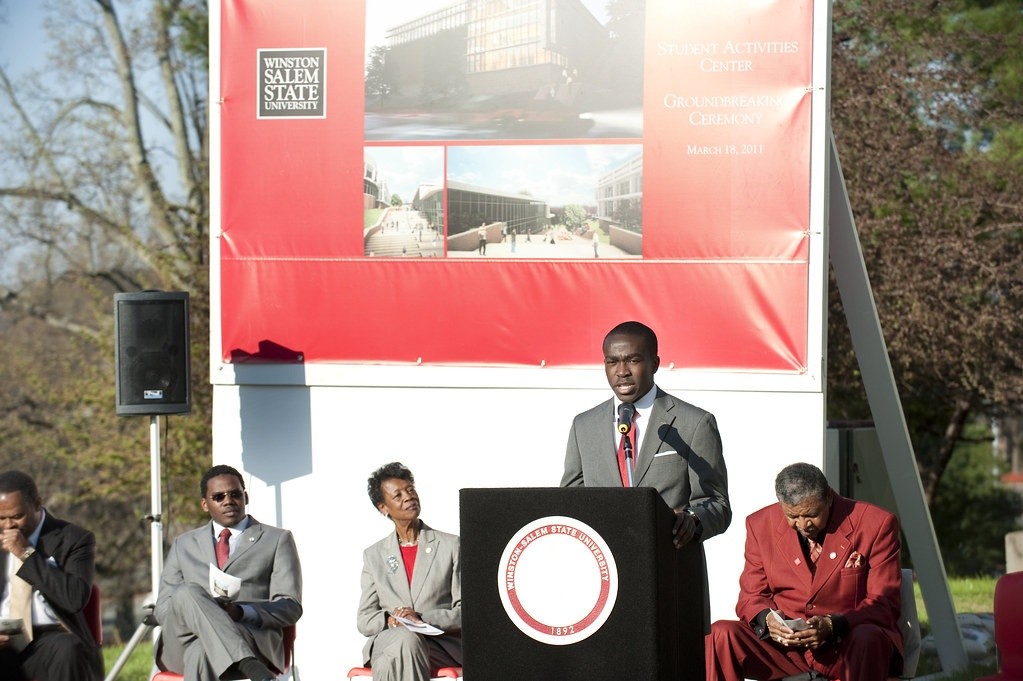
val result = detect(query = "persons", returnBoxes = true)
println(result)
[0,472,108,681]
[707,463,905,680]
[154,464,303,681]
[357,462,462,680]
[561,321,732,638]
[373,203,565,254]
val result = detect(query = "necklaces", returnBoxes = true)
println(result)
[396,537,419,547]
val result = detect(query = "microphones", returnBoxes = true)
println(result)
[618,401,636,434]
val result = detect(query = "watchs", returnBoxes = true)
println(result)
[20,549,36,562]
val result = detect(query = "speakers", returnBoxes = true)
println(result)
[114,292,191,416]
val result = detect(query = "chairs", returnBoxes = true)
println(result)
[348,665,463,681]
[141,602,296,680]
[971,569,1023,680]
[82,586,103,653]
[778,569,921,680]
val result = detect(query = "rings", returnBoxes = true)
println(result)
[398,606,403,612]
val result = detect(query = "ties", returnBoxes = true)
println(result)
[216,529,232,570]
[617,421,636,487]
[808,537,823,576]
[9,555,33,654]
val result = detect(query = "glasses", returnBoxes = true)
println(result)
[206,489,246,502]
